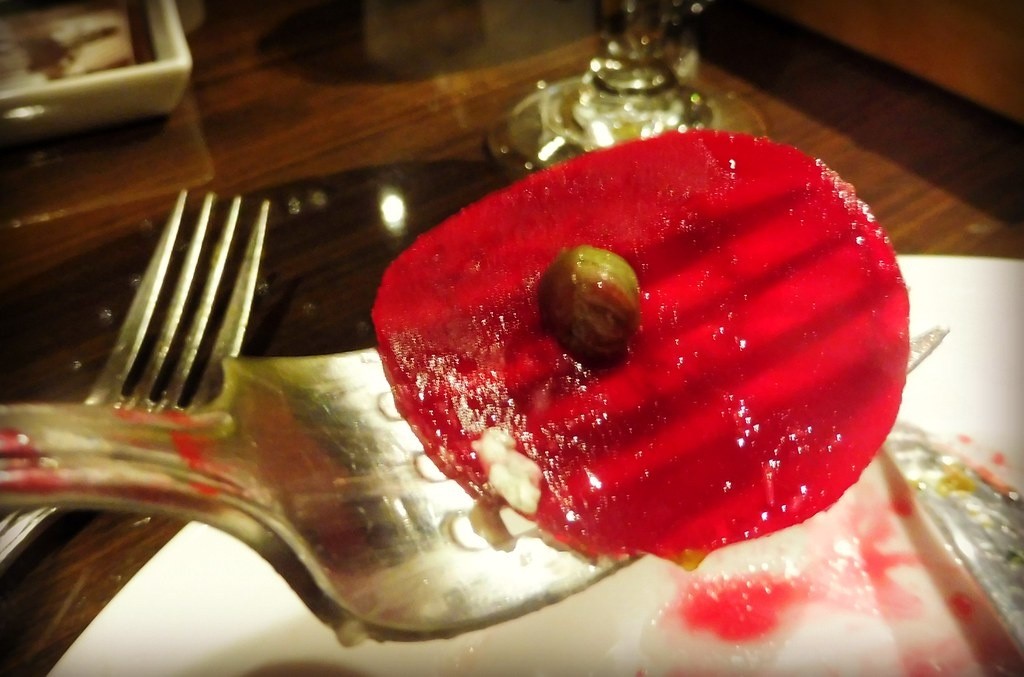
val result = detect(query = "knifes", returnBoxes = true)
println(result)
[877,422,1024,656]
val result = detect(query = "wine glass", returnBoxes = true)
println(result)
[482,0,775,185]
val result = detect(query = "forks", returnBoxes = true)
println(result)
[0,190,272,576]
[0,184,949,644]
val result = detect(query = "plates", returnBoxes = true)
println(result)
[44,254,1024,677]
